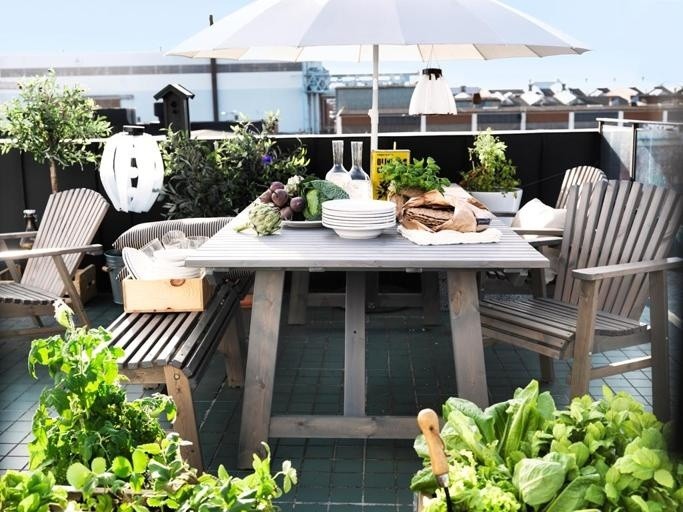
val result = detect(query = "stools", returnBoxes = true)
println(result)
[79,281,247,481]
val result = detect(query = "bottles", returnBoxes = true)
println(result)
[327,138,372,199]
[19,209,39,275]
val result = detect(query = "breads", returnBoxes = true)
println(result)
[386,184,454,229]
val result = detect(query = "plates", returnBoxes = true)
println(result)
[120,242,200,281]
[319,196,397,241]
[286,220,332,229]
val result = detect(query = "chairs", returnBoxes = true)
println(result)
[481,167,605,381]
[481,180,683,428]
[0,187,109,343]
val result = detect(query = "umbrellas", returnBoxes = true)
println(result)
[165,1,595,149]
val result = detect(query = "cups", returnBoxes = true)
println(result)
[140,230,213,271]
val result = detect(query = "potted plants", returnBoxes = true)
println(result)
[461,126,524,228]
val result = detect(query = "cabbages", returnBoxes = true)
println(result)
[292,176,350,222]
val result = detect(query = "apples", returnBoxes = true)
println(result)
[249,181,304,221]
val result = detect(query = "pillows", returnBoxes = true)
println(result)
[111,216,236,254]
[115,267,257,281]
